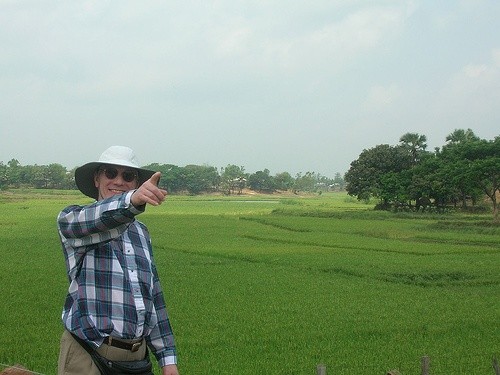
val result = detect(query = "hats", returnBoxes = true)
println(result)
[75,146,160,202]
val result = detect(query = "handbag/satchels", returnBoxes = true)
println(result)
[64,326,152,375]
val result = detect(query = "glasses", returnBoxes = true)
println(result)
[101,167,138,182]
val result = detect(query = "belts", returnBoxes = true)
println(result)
[103,336,144,352]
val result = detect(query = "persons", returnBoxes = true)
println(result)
[56,146,179,375]
